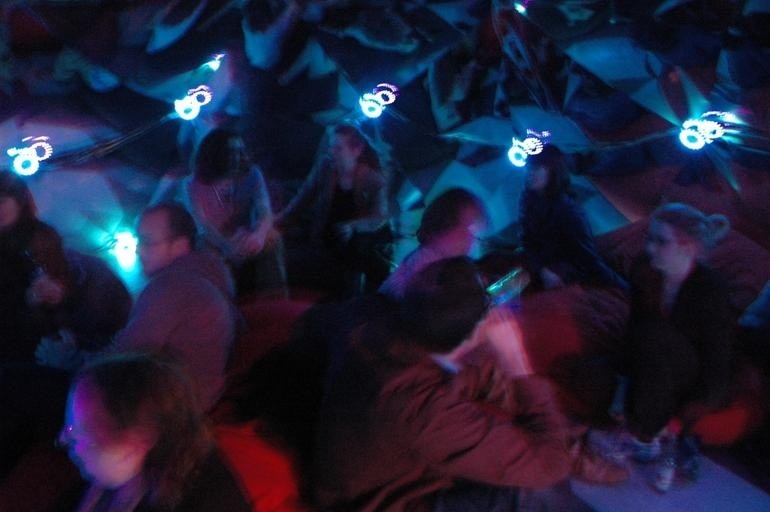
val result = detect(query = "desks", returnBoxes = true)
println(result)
[567,442,770,511]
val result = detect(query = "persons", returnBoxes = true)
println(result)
[0,122,745,512]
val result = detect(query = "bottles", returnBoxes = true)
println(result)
[484,266,530,310]
[649,433,678,494]
